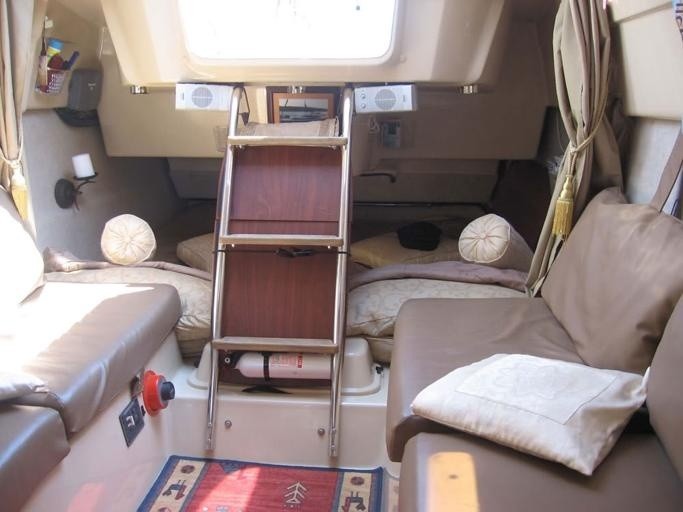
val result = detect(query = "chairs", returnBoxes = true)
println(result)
[384,184,680,464]
[0,182,185,438]
[0,396,72,512]
[398,293,681,512]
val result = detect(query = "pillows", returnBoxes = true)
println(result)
[457,213,532,273]
[96,212,157,266]
[408,350,652,480]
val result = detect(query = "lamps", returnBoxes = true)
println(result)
[54,151,98,212]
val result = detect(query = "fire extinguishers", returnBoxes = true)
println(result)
[224,354,332,379]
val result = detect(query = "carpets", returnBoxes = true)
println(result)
[135,455,386,512]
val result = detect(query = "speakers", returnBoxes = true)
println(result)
[354,84,412,114]
[176,83,233,111]
